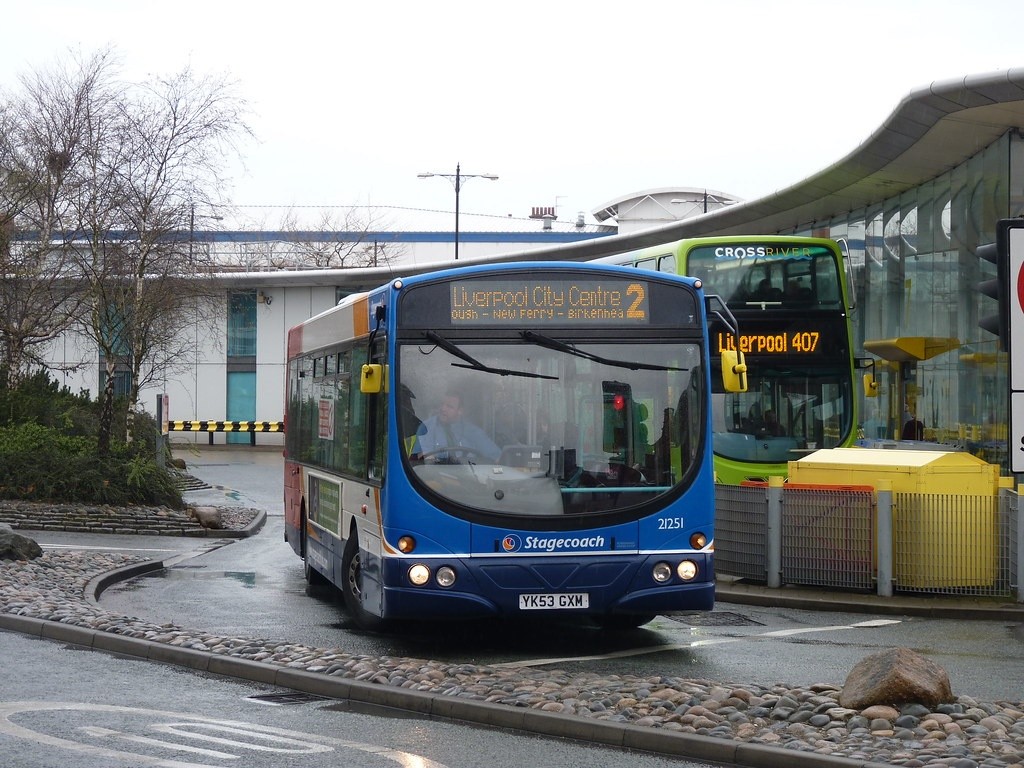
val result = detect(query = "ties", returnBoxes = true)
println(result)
[445,424,456,464]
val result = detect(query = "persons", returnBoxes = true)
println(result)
[765,411,786,438]
[901,417,923,441]
[410,386,502,464]
[748,277,812,303]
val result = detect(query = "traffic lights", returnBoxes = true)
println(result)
[602,380,632,453]
[973,217,1010,350]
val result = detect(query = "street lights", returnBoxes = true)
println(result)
[671,189,737,213]
[416,162,500,261]
[161,211,224,271]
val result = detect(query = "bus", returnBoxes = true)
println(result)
[282,263,749,656]
[572,233,880,487]
